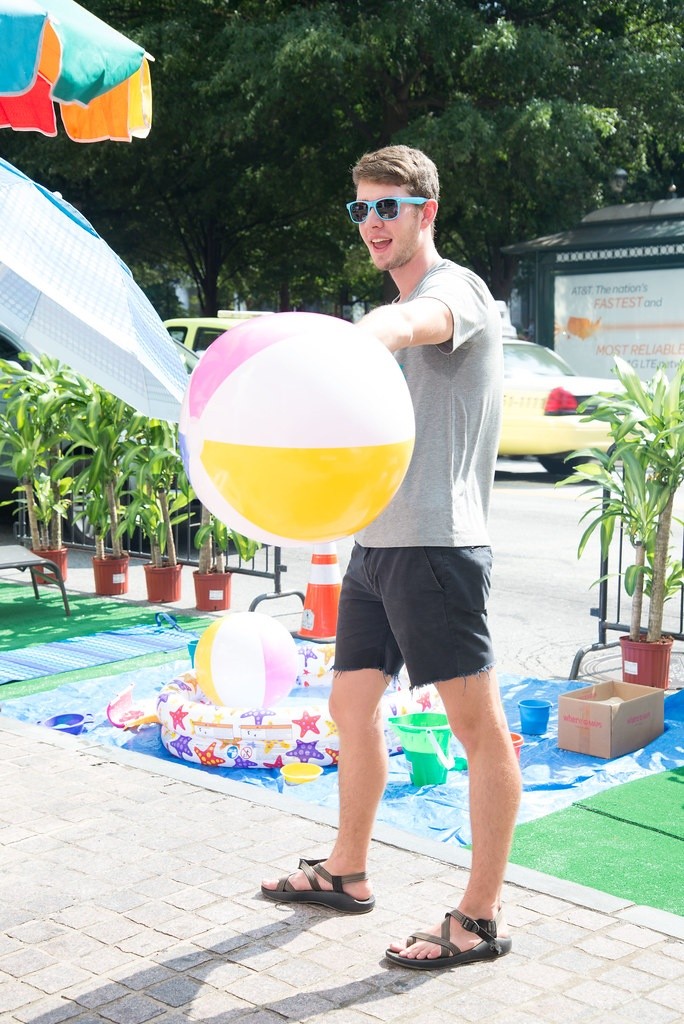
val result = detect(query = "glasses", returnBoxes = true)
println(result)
[345,197,429,224]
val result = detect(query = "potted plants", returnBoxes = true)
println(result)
[553,354,684,692]
[0,352,262,612]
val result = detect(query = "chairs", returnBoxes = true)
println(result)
[1,544,71,617]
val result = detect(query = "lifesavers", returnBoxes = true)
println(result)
[156,641,453,771]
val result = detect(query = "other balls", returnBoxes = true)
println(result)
[192,614,303,709]
[175,312,418,550]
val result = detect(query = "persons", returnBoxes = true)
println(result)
[262,144,523,971]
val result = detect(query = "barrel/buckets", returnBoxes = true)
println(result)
[508,732,524,760]
[390,711,456,787]
[518,700,552,735]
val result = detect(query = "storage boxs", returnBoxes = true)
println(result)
[558,680,665,759]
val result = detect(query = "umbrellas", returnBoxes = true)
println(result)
[0,0,157,144]
[0,157,190,423]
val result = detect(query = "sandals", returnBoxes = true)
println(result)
[385,910,512,970]
[261,858,375,914]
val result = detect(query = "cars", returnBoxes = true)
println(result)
[496,323,647,477]
[162,311,278,362]
